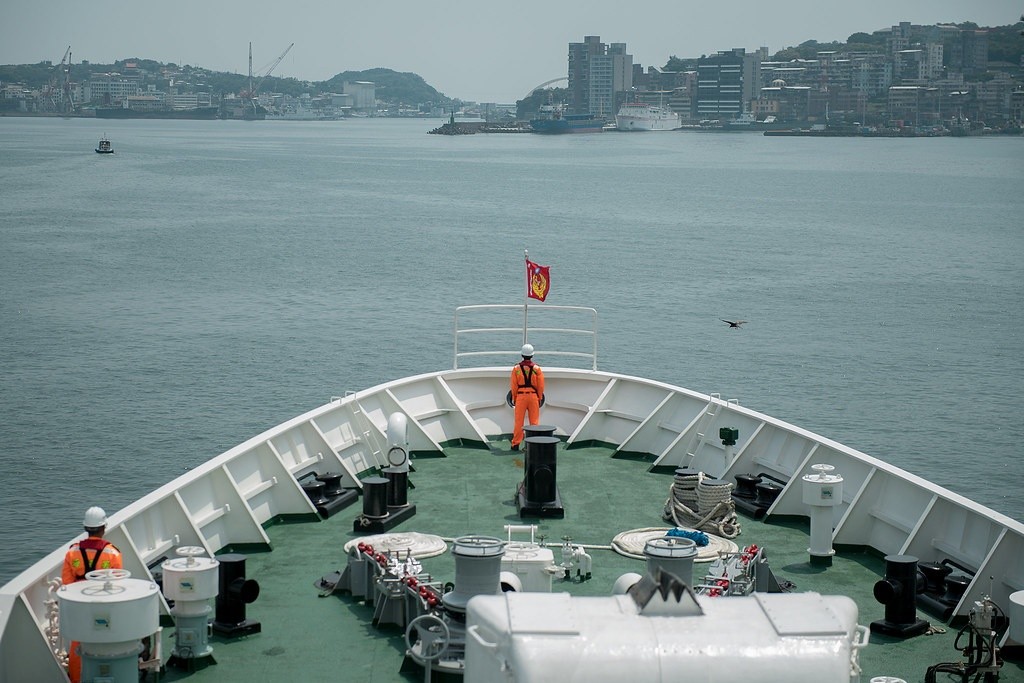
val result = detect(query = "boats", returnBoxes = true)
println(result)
[613,82,683,130]
[529,85,605,132]
[730,99,763,125]
[94,130,115,153]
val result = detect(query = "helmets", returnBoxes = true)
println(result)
[521,344,535,356]
[83,506,108,527]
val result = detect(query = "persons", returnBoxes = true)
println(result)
[510,338,544,451]
[61,505,123,683]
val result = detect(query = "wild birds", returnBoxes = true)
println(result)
[719,318,749,330]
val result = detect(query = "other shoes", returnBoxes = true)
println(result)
[510,444,519,451]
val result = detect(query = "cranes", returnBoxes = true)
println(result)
[234,41,295,114]
[43,46,74,111]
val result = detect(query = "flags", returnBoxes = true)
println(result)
[526,259,551,302]
